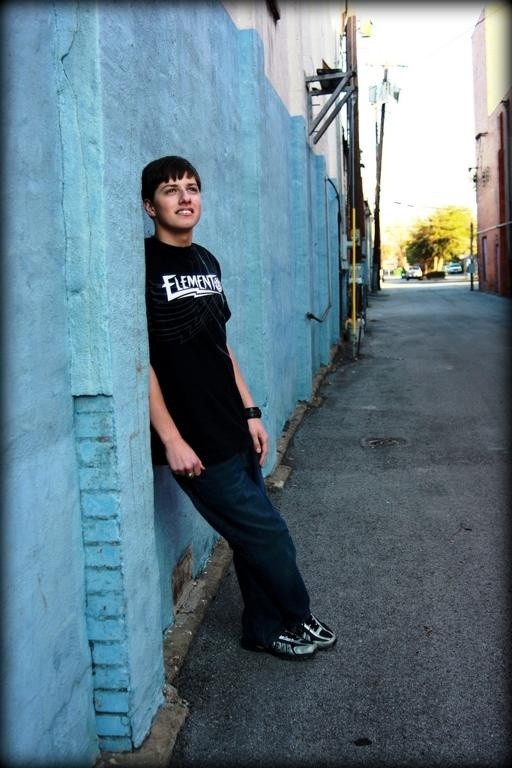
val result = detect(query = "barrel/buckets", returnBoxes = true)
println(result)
[348,322,365,342]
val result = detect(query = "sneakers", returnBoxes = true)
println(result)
[290,613,338,649]
[239,630,315,661]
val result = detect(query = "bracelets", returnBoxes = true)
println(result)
[243,405,261,422]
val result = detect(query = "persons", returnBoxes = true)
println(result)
[139,157,337,659]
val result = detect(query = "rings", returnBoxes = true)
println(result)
[187,471,195,477]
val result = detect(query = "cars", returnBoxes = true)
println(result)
[448,262,463,273]
[406,265,423,280]
